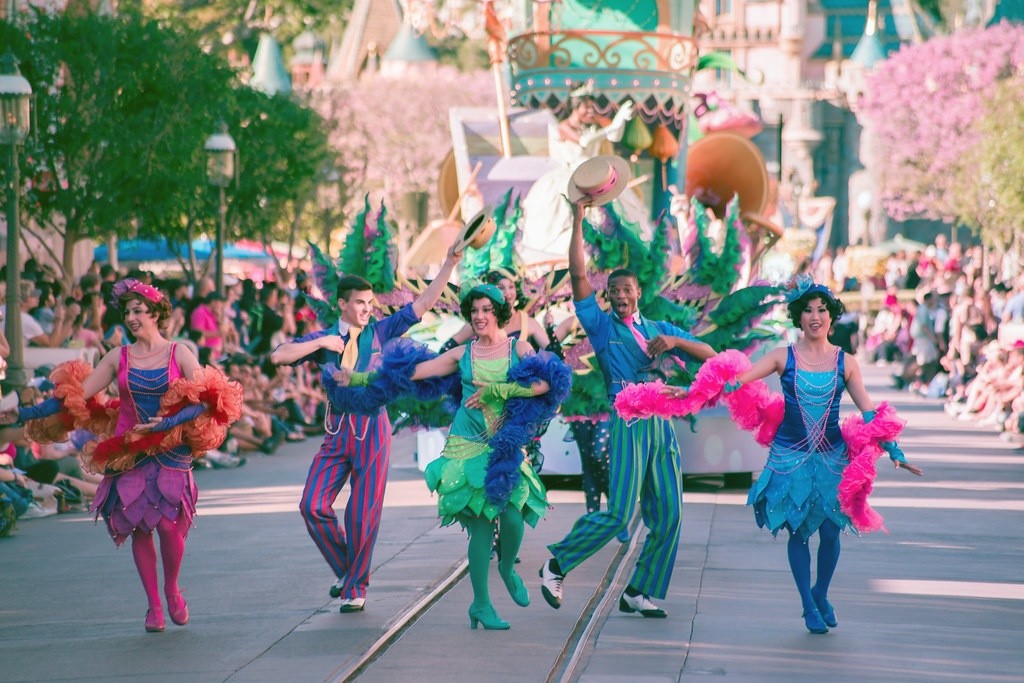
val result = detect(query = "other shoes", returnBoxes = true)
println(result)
[801,607,828,633]
[811,588,837,627]
[145,607,164,632]
[263,431,284,452]
[617,527,629,542]
[18,503,56,519]
[164,585,188,626]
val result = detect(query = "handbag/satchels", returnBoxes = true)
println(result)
[54,477,83,514]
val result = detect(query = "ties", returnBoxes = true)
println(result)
[622,314,654,360]
[340,326,362,370]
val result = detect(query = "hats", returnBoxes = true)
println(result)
[568,155,631,207]
[454,203,497,254]
[21,279,42,300]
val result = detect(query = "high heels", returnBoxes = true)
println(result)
[469,602,510,629]
[498,562,530,607]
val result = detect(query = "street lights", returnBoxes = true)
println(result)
[0,50,32,402]
[203,130,237,302]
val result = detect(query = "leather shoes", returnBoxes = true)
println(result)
[539,559,564,609]
[619,591,667,618]
[329,575,346,598]
[340,597,365,613]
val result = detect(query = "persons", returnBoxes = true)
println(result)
[0,232,1024,541]
[332,283,572,629]
[538,196,718,619]
[664,272,928,634]
[271,238,466,613]
[0,277,244,632]
[514,79,655,267]
